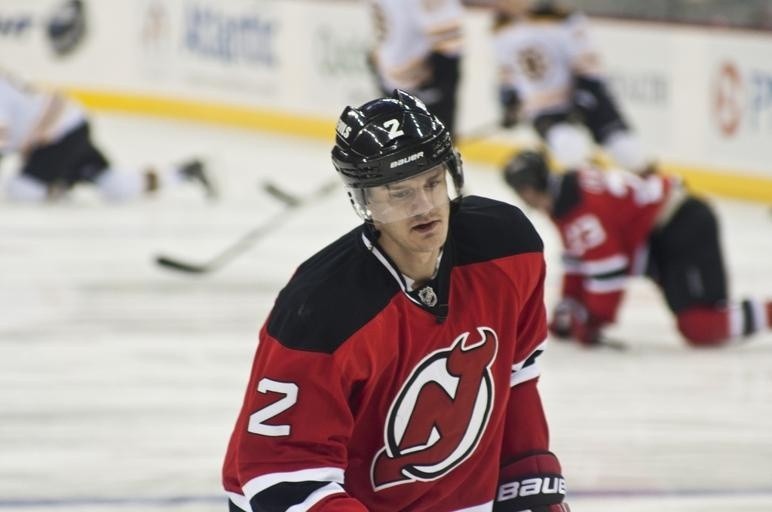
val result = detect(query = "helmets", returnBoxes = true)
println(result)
[505,150,547,191]
[330,87,464,209]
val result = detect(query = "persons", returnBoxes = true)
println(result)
[220,87,573,512]
[488,0,657,177]
[502,147,772,348]
[0,69,219,205]
[366,0,472,137]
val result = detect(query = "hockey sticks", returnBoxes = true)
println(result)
[157,175,344,274]
[259,172,346,209]
